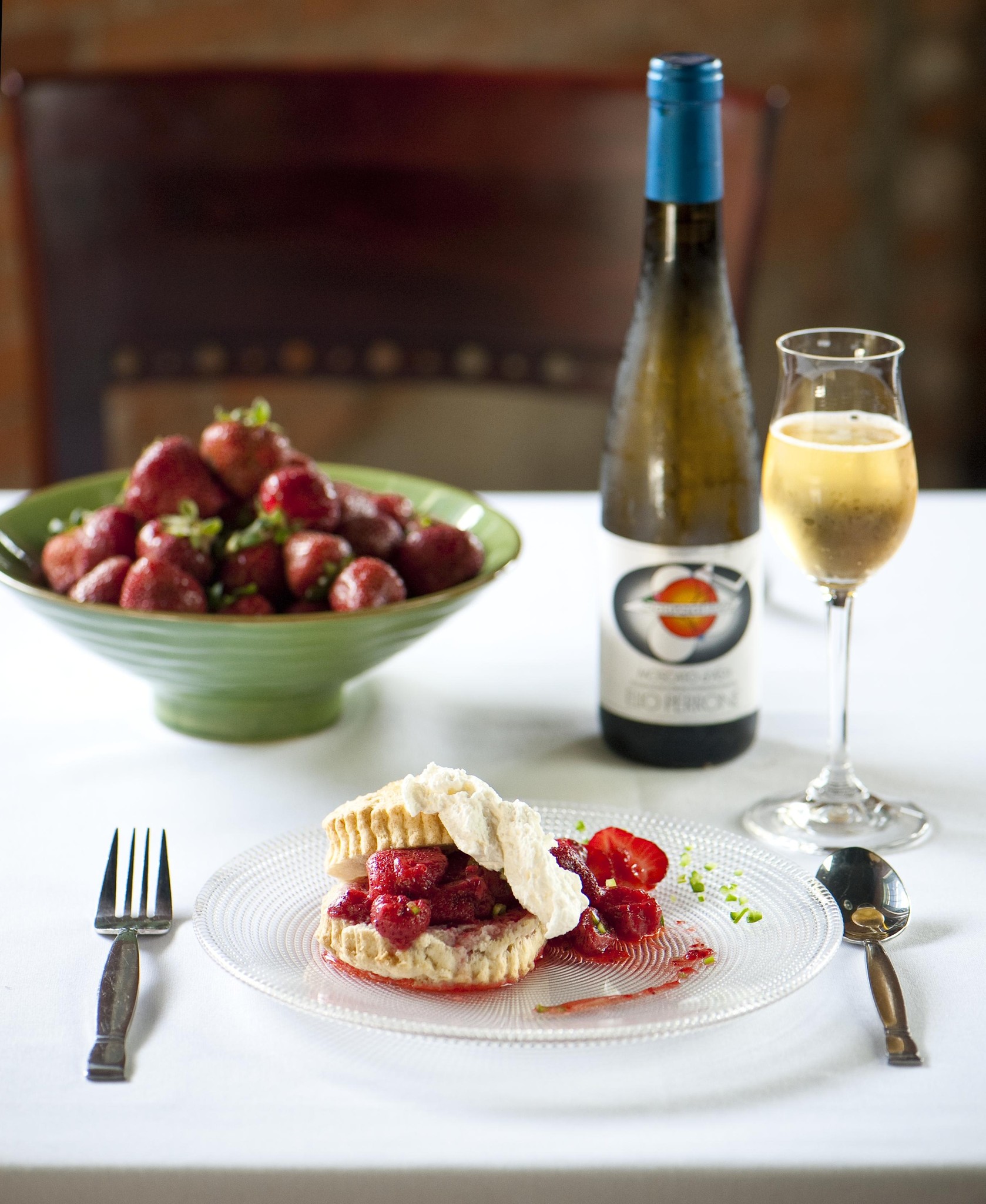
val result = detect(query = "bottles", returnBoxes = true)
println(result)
[599,52,764,770]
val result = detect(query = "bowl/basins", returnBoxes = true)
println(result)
[0,457,520,744]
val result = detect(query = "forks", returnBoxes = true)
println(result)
[85,828,174,1082]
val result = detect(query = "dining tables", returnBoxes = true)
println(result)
[0,490,981,1204]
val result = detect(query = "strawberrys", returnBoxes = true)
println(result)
[39,403,480,616]
[550,828,671,955]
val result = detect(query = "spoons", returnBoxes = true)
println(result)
[812,845,923,1069]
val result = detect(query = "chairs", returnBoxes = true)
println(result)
[16,65,787,490]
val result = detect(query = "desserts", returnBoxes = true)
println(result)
[315,761,590,990]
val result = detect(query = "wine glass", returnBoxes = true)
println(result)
[739,327,936,858]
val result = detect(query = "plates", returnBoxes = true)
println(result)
[191,804,846,1047]
